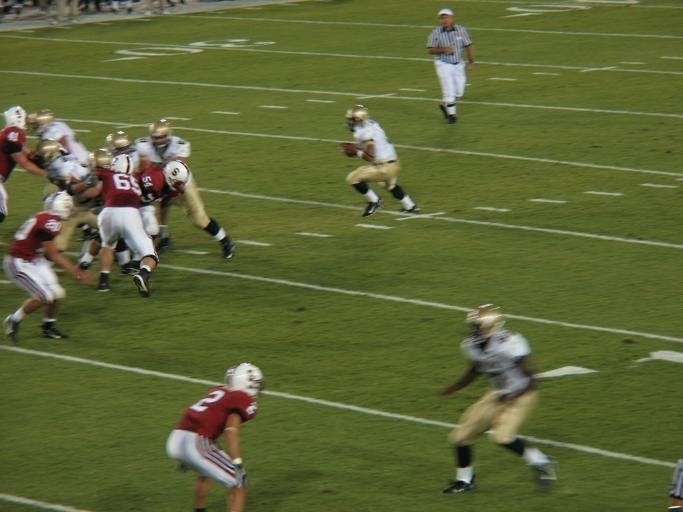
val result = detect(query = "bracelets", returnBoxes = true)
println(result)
[469,56,476,65]
[355,148,365,160]
[231,456,243,468]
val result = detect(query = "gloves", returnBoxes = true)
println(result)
[236,463,248,488]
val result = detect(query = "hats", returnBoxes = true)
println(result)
[437,8,453,16]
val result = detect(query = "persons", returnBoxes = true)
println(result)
[0,0,185,19]
[664,457,683,511]
[337,102,422,220]
[162,358,267,512]
[426,300,557,497]
[425,8,477,128]
[0,106,234,346]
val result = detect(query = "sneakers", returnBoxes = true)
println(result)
[3,314,18,344]
[40,319,66,338]
[77,238,169,297]
[362,197,383,217]
[443,474,476,494]
[400,207,420,213]
[449,114,457,124]
[223,239,236,259]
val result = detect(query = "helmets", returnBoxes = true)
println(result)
[466,304,503,340]
[4,106,190,191]
[44,191,75,219]
[345,104,368,126]
[225,363,263,397]
